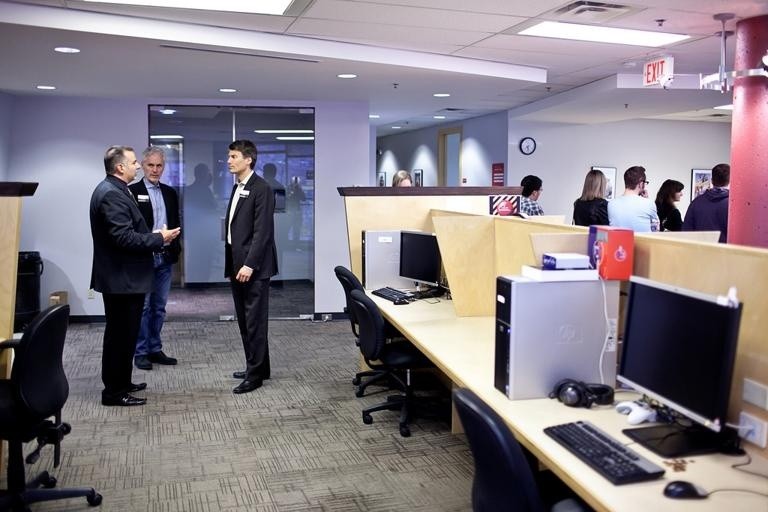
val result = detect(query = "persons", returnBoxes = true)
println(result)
[128,148,181,371]
[260,162,308,289]
[391,169,414,189]
[573,169,609,225]
[88,144,182,408]
[184,160,222,293]
[521,173,545,217]
[683,163,730,244]
[655,178,685,233]
[223,141,280,395]
[607,166,661,233]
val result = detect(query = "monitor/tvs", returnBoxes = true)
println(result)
[615,275,742,459]
[397,230,441,298]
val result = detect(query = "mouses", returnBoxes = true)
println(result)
[663,481,708,499]
[393,297,408,305]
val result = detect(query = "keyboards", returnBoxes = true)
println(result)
[371,286,415,301]
[543,421,664,486]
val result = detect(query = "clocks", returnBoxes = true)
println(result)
[520,137,536,155]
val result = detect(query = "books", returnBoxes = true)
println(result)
[521,263,599,283]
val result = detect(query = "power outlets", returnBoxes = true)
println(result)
[738,412,767,448]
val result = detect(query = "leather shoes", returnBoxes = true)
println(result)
[149,350,177,365]
[135,355,153,370]
[102,384,147,405]
[233,372,263,393]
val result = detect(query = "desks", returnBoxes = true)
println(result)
[364,288,768,512]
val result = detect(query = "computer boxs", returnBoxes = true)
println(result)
[361,230,432,290]
[494,275,620,401]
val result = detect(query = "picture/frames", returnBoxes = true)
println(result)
[592,166,617,201]
[691,169,714,203]
[413,169,423,187]
[375,172,386,188]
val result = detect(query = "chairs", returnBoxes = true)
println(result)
[350,289,451,437]
[334,266,408,385]
[453,386,585,512]
[0,303,103,512]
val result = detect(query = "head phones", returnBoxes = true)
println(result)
[548,379,613,408]
